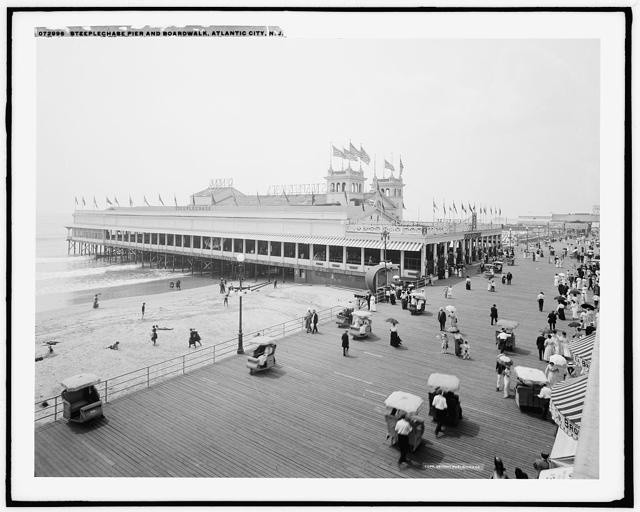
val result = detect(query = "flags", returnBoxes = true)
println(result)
[75,190,349,205]
[382,202,385,212]
[433,202,501,216]
[403,201,407,209]
[361,202,365,211]
[376,182,382,197]
[333,142,404,175]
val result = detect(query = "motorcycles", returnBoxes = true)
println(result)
[240,285,428,374]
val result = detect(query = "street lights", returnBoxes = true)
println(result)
[526,226,529,250]
[228,276,248,353]
[509,227,512,250]
[381,227,390,288]
[538,225,541,243]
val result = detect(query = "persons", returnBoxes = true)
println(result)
[35,278,276,367]
[303,242,600,478]
